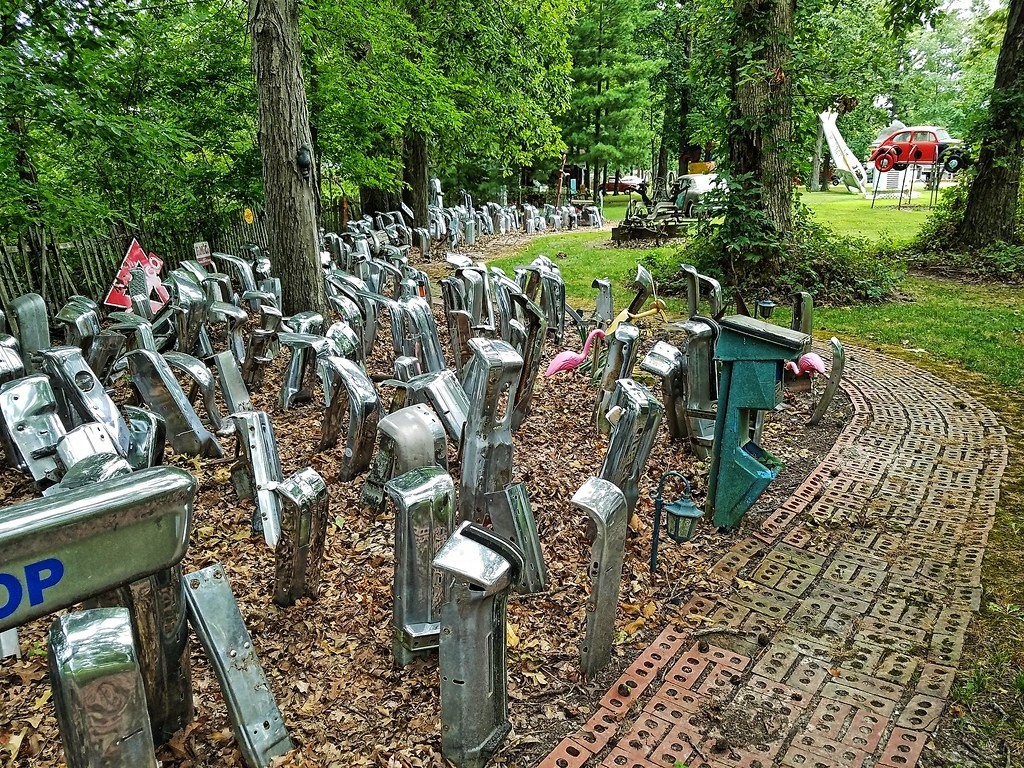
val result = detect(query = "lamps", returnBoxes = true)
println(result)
[648,472,705,548]
[754,286,775,320]
[534,216,542,231]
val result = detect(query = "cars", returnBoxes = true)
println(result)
[869,126,972,172]
[675,173,731,219]
[598,175,643,195]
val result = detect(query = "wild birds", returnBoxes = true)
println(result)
[545,329,606,378]
[785,352,826,413]
